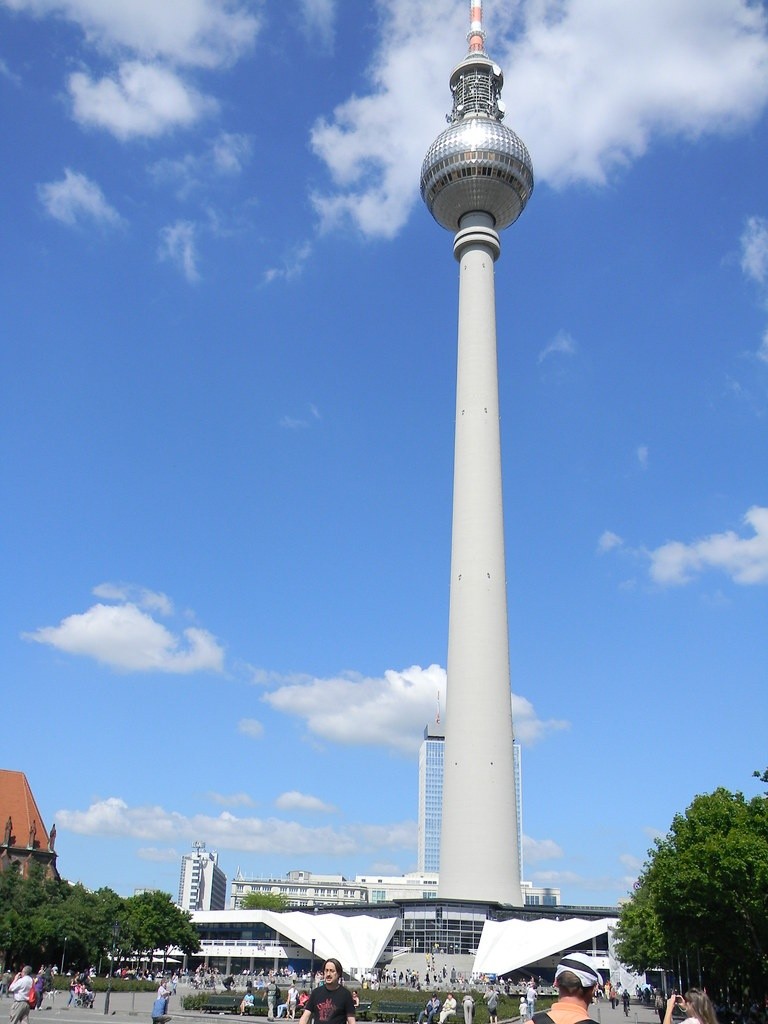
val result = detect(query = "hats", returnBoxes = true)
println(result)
[432,993,437,997]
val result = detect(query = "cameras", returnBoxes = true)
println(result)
[675,997,683,1003]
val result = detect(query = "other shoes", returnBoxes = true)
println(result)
[267,1017,274,1022]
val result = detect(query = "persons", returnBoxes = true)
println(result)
[361,944,554,1024]
[299,958,356,1024]
[711,994,768,1024]
[523,952,603,1024]
[346,990,359,1024]
[0,942,344,1024]
[663,987,719,1024]
[590,980,684,1024]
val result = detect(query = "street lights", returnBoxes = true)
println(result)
[61,937,67,975]
[104,919,123,1014]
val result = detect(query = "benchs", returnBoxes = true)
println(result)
[203,995,476,1024]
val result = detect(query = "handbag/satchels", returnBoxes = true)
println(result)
[29,978,36,1009]
[296,995,300,1004]
[427,1005,432,1013]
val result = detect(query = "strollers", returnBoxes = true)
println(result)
[75,985,94,1008]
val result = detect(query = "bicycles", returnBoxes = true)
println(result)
[624,1000,630,1016]
[188,978,207,990]
